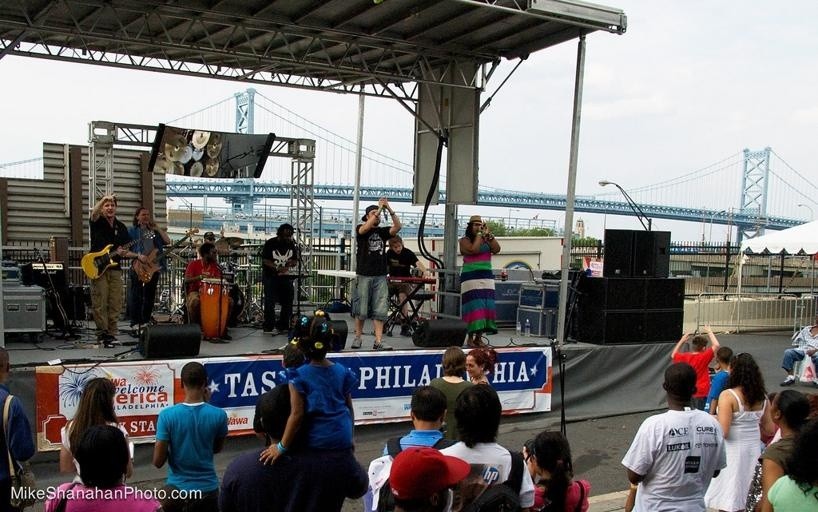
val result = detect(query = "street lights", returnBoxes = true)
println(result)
[796,202,814,222]
[599,180,653,232]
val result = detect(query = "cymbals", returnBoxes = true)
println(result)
[239,244,263,248]
[182,242,201,247]
[152,130,225,176]
[214,236,243,254]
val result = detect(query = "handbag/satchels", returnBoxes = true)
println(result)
[10,462,37,508]
[473,485,521,512]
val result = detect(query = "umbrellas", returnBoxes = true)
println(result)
[742,220,817,325]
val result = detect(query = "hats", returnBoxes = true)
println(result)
[367,454,394,511]
[467,214,483,226]
[389,445,472,501]
[361,205,379,222]
[204,232,214,238]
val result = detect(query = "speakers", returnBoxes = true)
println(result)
[413,319,468,346]
[602,229,671,277]
[582,277,686,344]
[140,325,202,356]
[329,320,347,348]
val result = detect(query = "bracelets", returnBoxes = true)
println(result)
[373,213,377,219]
[476,231,483,236]
[390,212,395,216]
[485,233,495,240]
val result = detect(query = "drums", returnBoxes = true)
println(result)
[198,278,230,340]
[228,284,244,322]
[238,264,261,271]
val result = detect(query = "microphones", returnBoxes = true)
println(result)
[481,223,487,228]
[382,206,389,220]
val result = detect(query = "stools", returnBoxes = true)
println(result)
[400,290,434,321]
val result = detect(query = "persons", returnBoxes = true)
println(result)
[0,310,589,512]
[460,215,500,347]
[622,323,818,512]
[185,243,232,340]
[351,196,402,350]
[89,194,129,344]
[385,235,426,337]
[261,224,298,333]
[127,207,170,337]
[196,232,216,261]
[591,255,603,276]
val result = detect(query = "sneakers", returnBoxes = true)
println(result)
[400,329,411,336]
[781,378,795,386]
[351,336,362,348]
[373,340,392,350]
[97,333,116,342]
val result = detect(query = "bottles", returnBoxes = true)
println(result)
[516,321,521,338]
[524,318,531,337]
[492,266,509,281]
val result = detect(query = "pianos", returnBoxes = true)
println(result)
[385,276,439,285]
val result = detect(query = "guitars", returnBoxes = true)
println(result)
[81,231,155,279]
[131,227,199,283]
[34,248,71,334]
